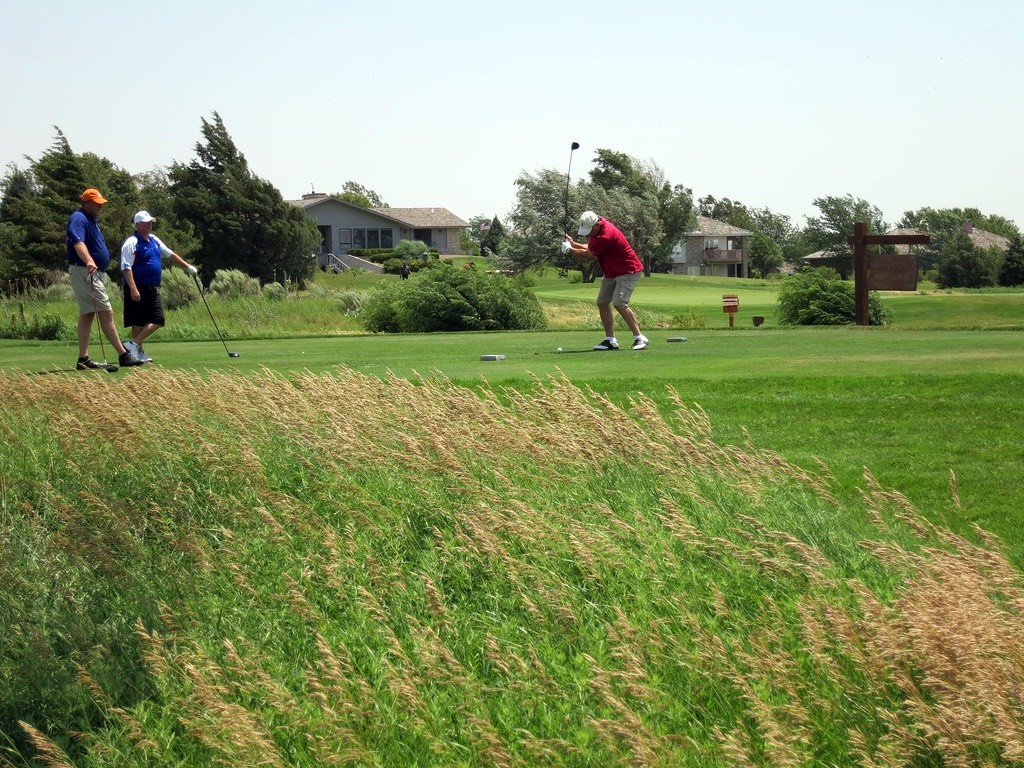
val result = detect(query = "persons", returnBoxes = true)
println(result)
[400,261,412,282]
[67,189,145,372]
[562,212,651,352]
[118,210,198,364]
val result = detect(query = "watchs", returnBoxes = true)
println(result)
[566,247,572,257]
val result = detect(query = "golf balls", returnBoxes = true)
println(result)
[557,348,563,353]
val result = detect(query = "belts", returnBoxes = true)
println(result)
[73,262,109,274]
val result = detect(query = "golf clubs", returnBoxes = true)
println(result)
[192,273,240,357]
[89,272,118,373]
[564,142,580,243]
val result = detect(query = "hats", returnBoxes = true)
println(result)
[134,210,156,224]
[81,188,108,205]
[578,211,597,236]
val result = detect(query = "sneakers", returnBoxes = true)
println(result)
[118,341,153,367]
[595,337,619,351]
[76,356,107,370]
[632,334,649,350]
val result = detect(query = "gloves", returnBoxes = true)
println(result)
[561,241,571,255]
[184,265,197,277]
